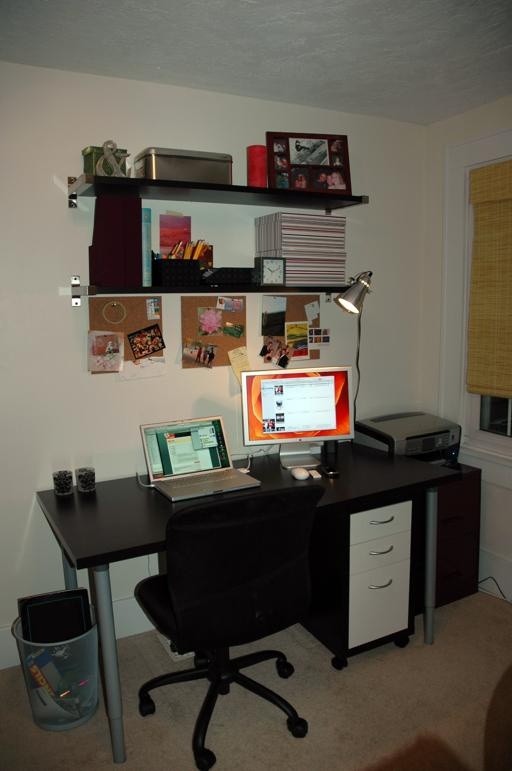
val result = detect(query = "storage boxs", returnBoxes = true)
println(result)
[256,248,347,287]
[252,212,346,251]
[133,146,233,186]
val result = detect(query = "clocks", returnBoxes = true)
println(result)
[254,257,286,288]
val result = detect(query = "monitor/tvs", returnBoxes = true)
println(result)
[241,366,354,469]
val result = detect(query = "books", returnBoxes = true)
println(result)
[150,239,208,261]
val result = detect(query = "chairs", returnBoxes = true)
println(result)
[129,482,327,771]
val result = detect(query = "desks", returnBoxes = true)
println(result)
[32,439,463,771]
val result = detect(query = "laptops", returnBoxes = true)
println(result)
[140,416,261,502]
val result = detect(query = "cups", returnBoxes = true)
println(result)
[75,457,97,494]
[50,457,74,495]
[246,145,269,189]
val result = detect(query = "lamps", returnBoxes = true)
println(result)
[336,269,372,427]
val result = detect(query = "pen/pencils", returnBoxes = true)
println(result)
[168,239,205,261]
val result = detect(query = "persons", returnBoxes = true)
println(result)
[271,135,345,190]
[233,301,241,309]
[201,350,207,364]
[132,328,162,355]
[219,298,230,308]
[106,341,113,361]
[263,386,284,431]
[260,341,288,367]
[195,347,202,363]
[207,347,214,368]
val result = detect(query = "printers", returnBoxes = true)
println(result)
[352,411,462,466]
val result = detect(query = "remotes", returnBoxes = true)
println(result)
[309,470,321,478]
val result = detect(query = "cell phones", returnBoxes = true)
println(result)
[319,465,340,477]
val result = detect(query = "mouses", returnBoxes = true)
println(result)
[291,467,310,480]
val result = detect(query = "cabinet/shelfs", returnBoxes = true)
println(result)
[66,174,369,297]
[413,462,481,616]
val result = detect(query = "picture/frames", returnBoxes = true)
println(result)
[265,130,353,196]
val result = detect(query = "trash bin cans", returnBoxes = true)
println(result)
[10,613,101,731]
